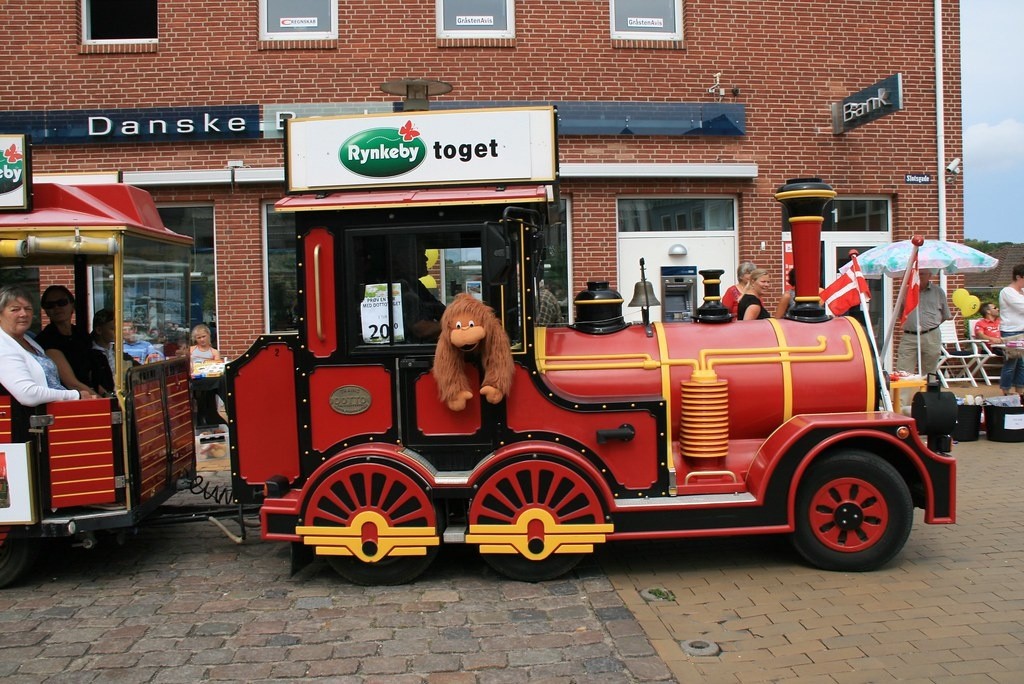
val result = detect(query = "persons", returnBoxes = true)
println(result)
[0,282,116,407]
[187,325,223,429]
[895,269,951,407]
[974,302,1005,356]
[527,280,563,328]
[999,264,1024,396]
[122,320,165,365]
[148,324,190,353]
[344,238,447,349]
[722,262,796,321]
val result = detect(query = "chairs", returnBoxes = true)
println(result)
[958,318,1009,385]
[939,321,996,389]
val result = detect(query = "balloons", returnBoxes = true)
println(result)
[952,288,980,317]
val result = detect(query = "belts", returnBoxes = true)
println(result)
[904,325,939,335]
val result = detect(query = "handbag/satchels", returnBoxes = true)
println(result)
[1001,333,1024,359]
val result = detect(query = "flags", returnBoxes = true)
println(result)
[820,263,872,317]
[901,249,920,329]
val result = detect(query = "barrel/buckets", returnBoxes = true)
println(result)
[951,405,982,441]
[982,400,1024,443]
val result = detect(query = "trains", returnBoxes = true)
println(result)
[0,105,958,586]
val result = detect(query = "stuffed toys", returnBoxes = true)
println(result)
[433,293,515,412]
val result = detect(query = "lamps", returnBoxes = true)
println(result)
[669,244,686,256]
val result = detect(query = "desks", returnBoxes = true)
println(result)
[185,359,225,429]
[888,374,927,414]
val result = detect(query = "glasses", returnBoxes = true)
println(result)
[123,326,136,331]
[44,298,68,307]
[987,307,996,310]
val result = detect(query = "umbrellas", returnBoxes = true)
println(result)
[838,239,999,376]
